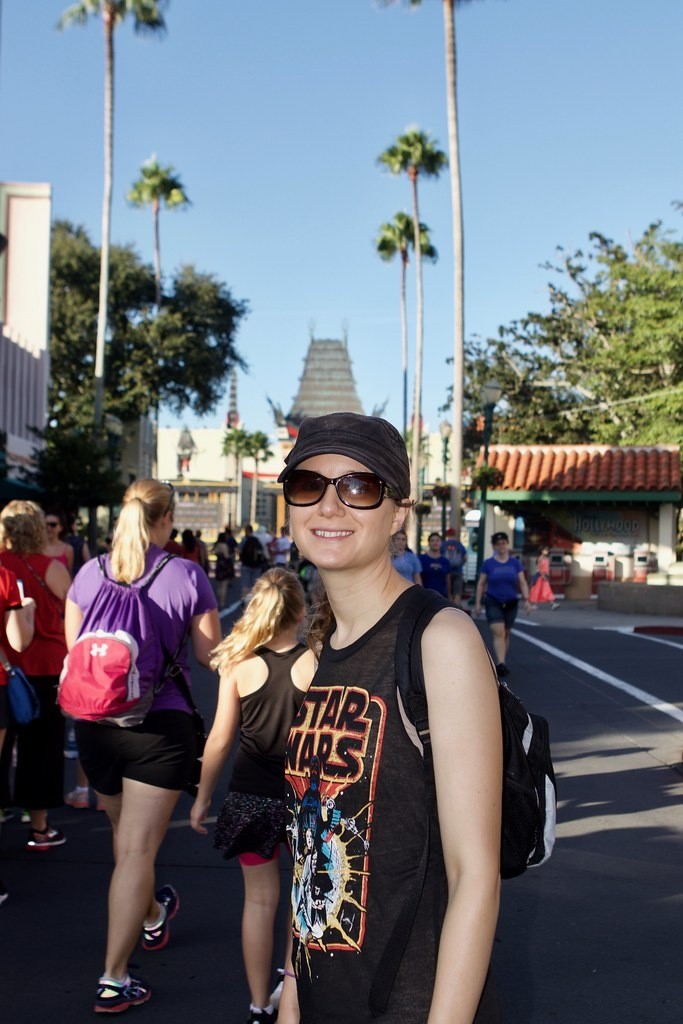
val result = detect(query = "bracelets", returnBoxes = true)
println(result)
[524,598,530,601]
[284,971,296,977]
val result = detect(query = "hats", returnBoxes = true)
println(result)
[491,532,507,545]
[277,413,412,499]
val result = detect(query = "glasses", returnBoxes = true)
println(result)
[46,521,61,528]
[282,469,394,510]
[161,482,174,517]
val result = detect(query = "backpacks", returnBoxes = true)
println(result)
[394,588,555,879]
[55,553,190,729]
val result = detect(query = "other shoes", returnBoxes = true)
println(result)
[496,663,509,678]
[0,808,14,823]
[62,739,79,759]
[64,786,89,807]
[92,789,123,812]
[25,826,67,851]
[247,1004,278,1024]
[21,808,49,822]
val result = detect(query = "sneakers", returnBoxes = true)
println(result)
[94,973,151,1013]
[140,884,179,951]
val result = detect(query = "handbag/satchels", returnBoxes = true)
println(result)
[7,668,39,722]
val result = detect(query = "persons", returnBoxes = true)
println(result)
[0,499,118,850]
[530,545,561,611]
[391,527,530,676]
[164,524,301,611]
[191,567,323,1024]
[64,479,222,1014]
[277,411,503,1024]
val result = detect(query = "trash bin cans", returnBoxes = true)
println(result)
[592,552,615,598]
[548,549,572,599]
[634,549,658,583]
